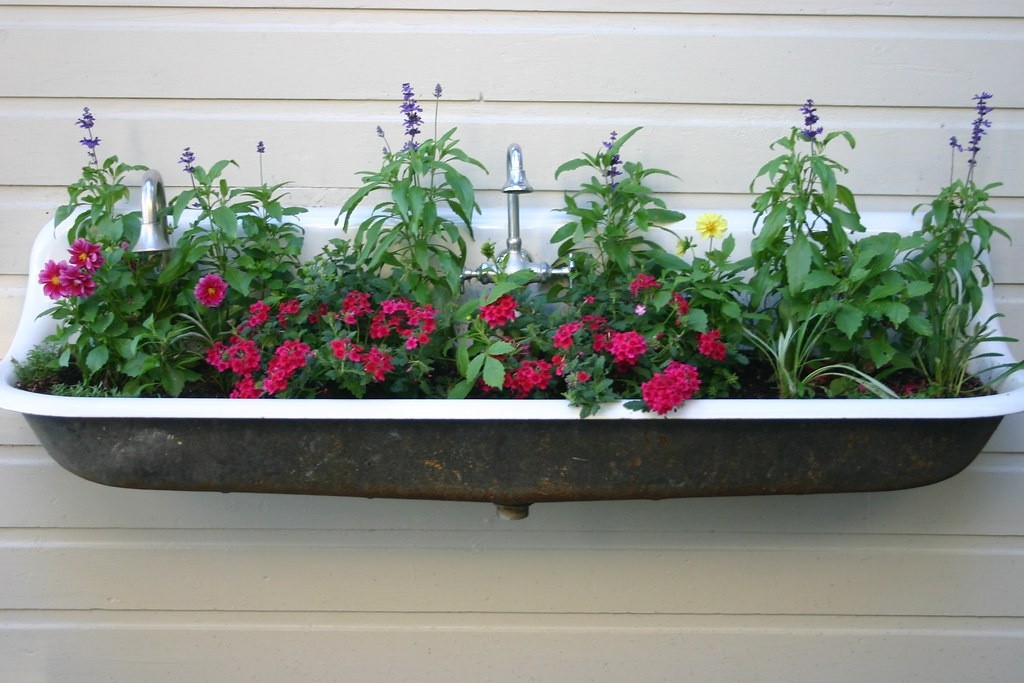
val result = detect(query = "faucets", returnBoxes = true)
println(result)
[129,168,173,257]
[497,142,536,254]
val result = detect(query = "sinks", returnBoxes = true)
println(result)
[0,207,1022,520]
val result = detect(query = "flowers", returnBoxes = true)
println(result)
[12,81,1024,419]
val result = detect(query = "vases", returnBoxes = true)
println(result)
[0,202,1024,523]
